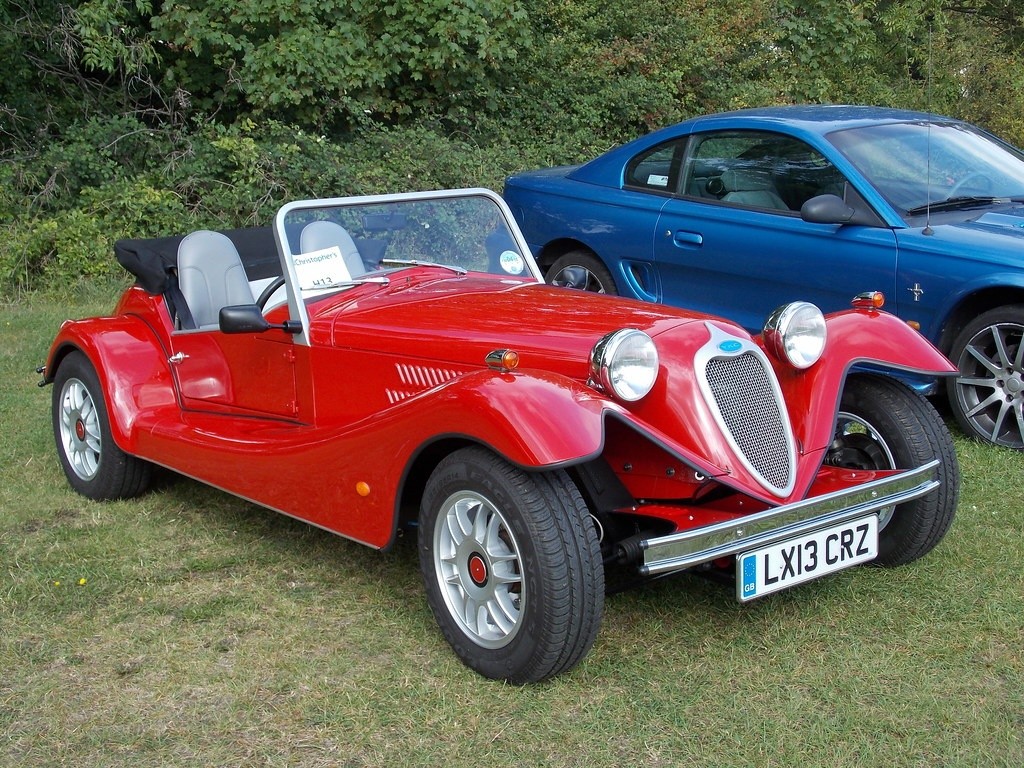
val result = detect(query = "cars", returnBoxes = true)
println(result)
[36,186,960,685]
[487,104,1024,452]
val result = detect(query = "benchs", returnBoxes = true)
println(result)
[637,155,830,211]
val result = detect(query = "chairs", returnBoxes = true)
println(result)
[177,229,255,330]
[719,169,789,209]
[815,164,864,198]
[299,221,366,277]
